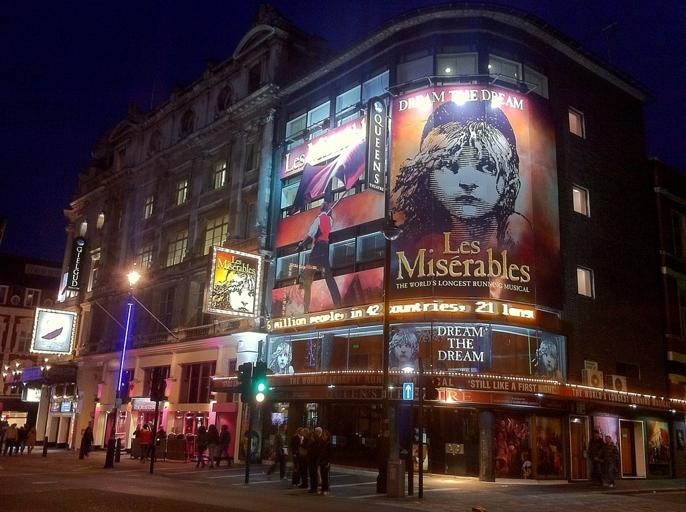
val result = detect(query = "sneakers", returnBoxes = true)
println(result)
[266,472,287,480]
[2,451,32,457]
[194,463,233,471]
[290,483,331,496]
[130,454,151,465]
[588,481,618,490]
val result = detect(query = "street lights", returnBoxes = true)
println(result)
[102,261,141,470]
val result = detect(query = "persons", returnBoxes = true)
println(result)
[532,342,562,378]
[269,341,295,376]
[388,327,419,371]
[132,420,167,464]
[372,429,389,494]
[195,425,208,468]
[595,435,620,488]
[215,424,232,466]
[0,420,37,456]
[292,187,342,308]
[220,265,255,316]
[395,97,540,264]
[77,426,94,460]
[586,428,606,488]
[605,434,620,468]
[266,422,331,495]
[205,424,220,468]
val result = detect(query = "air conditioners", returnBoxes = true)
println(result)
[581,368,603,389]
[605,374,627,393]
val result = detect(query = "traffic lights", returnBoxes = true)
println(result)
[253,361,268,404]
[150,366,166,401]
[232,361,253,404]
